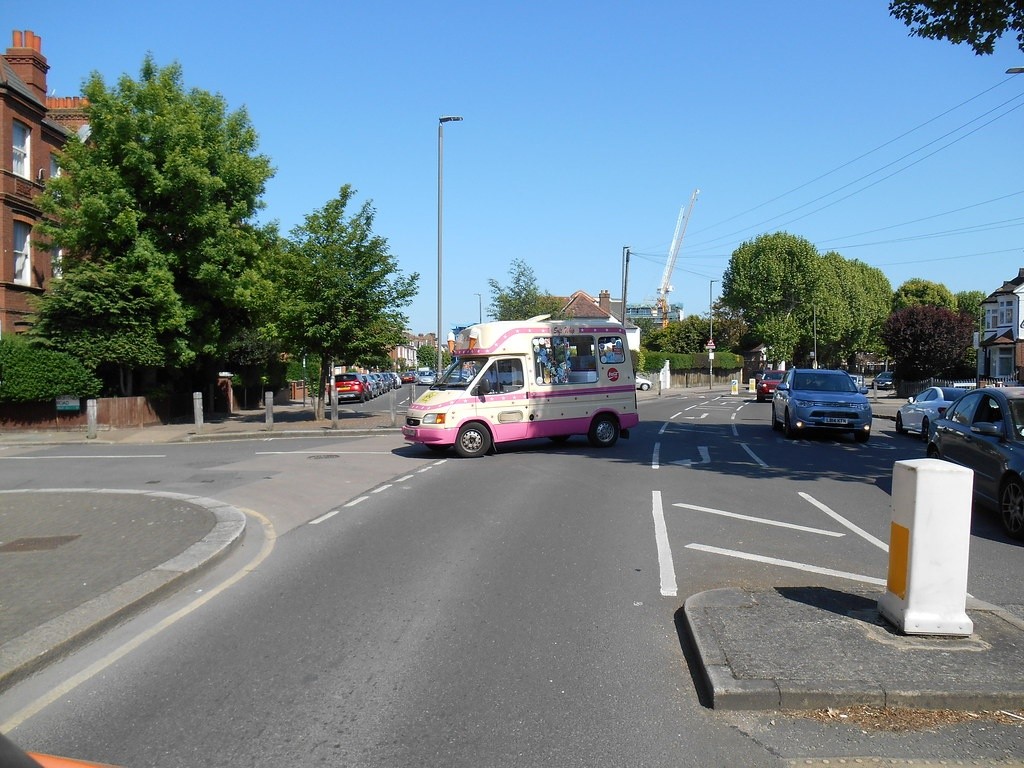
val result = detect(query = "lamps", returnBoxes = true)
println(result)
[37,168,46,184]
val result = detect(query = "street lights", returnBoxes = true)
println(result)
[710,280,717,390]
[437,116,464,380]
[474,293,482,324]
[621,246,628,323]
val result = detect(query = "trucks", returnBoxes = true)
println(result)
[402,319,639,458]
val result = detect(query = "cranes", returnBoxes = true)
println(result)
[655,189,698,330]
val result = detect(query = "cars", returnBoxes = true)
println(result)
[927,387,1024,539]
[369,373,401,395]
[418,371,437,385]
[896,387,999,443]
[636,376,652,391]
[872,372,897,390]
[756,370,788,401]
[400,371,419,384]
[328,373,370,404]
[847,374,866,389]
[362,375,378,399]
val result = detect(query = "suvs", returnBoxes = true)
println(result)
[771,368,872,443]
